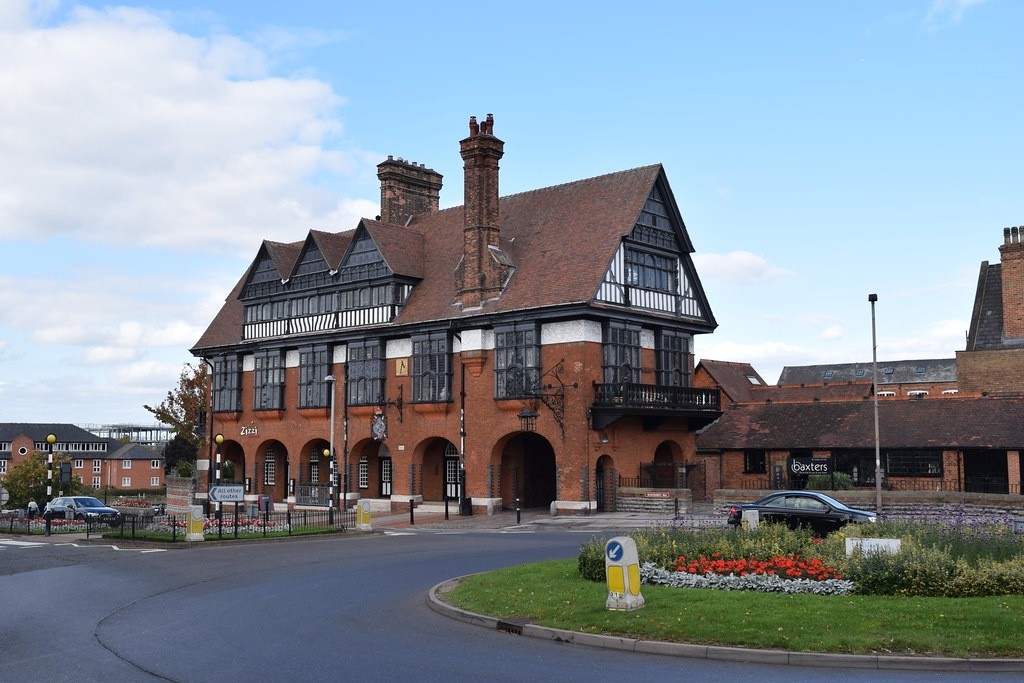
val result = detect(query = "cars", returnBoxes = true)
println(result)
[727,491,887,538]
[44,497,122,528]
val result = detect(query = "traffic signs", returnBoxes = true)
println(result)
[208,485,244,502]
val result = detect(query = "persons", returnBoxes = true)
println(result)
[18,504,24,523]
[26,497,39,521]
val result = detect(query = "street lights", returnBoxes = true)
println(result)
[321,448,334,525]
[213,433,224,519]
[45,432,57,536]
[869,293,884,516]
